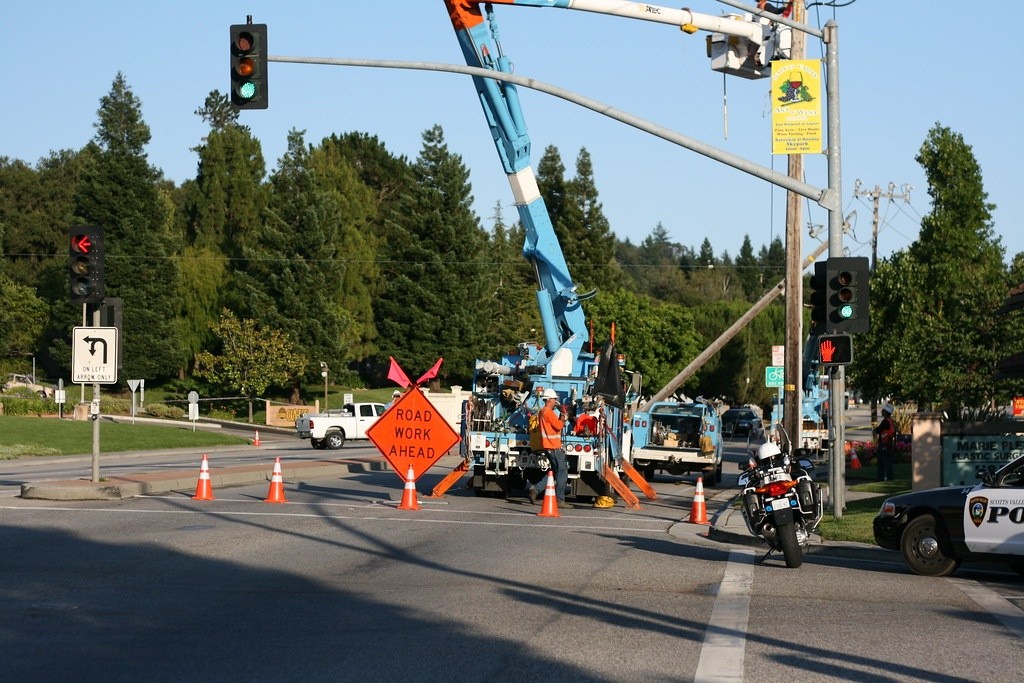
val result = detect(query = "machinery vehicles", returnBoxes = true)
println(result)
[425,1,794,509]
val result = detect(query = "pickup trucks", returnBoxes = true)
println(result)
[295,402,387,449]
[631,401,724,486]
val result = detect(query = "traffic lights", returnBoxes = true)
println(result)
[827,256,870,333]
[810,260,827,333]
[68,225,105,302]
[229,22,268,110]
[817,334,854,366]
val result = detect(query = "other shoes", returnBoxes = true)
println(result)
[557,500,574,508]
[529,488,538,505]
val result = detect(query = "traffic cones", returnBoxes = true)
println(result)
[191,454,215,500]
[536,471,561,517]
[682,477,712,524]
[263,458,289,503]
[396,463,423,509]
[253,430,261,446]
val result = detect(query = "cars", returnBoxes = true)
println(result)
[721,408,764,438]
[2,373,52,398]
[872,453,1024,577]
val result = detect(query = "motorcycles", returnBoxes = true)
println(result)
[738,423,823,569]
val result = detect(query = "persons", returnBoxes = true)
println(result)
[527,388,574,509]
[872,404,896,482]
[384,390,401,411]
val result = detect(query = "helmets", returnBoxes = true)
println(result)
[884,404,894,413]
[542,388,558,399]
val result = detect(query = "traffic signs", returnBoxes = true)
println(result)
[71,327,118,384]
